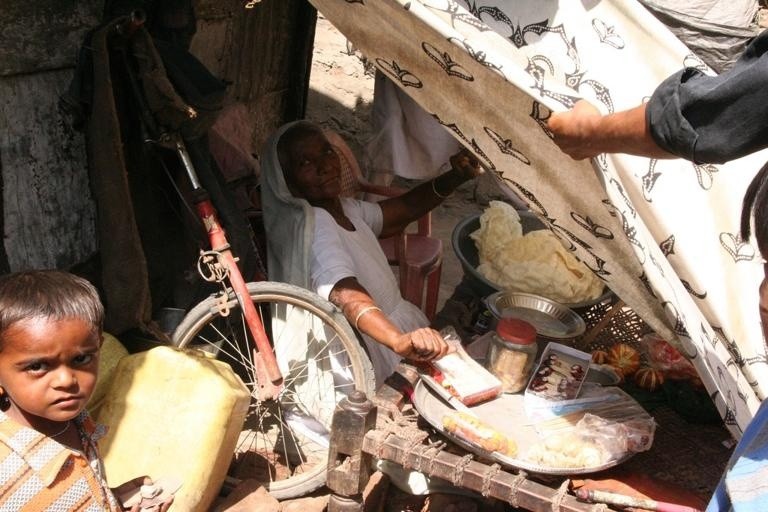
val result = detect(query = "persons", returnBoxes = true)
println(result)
[573,164,768,510]
[545,25,768,164]
[0,269,175,512]
[259,120,480,363]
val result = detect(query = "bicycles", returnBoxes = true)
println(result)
[144,113,378,500]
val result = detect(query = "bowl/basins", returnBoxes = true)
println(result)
[451,210,625,350]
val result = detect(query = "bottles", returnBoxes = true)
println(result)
[487,319,539,398]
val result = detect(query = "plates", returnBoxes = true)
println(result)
[587,366,619,393]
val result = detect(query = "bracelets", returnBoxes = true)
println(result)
[354,306,383,335]
[432,176,456,200]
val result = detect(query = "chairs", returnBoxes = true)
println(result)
[322,128,442,323]
